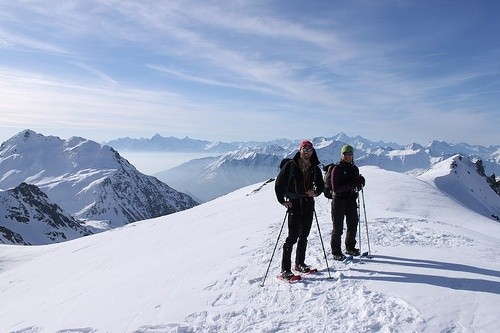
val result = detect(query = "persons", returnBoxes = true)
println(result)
[275,140,324,278]
[330,144,365,261]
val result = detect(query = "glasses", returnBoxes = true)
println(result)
[344,153,352,156]
[301,148,313,152]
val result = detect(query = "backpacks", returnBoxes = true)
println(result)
[322,162,357,199]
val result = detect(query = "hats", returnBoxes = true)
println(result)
[341,144,353,155]
[299,140,313,148]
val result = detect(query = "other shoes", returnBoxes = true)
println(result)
[347,249,360,258]
[333,254,346,262]
[277,271,294,280]
[294,265,310,272]
